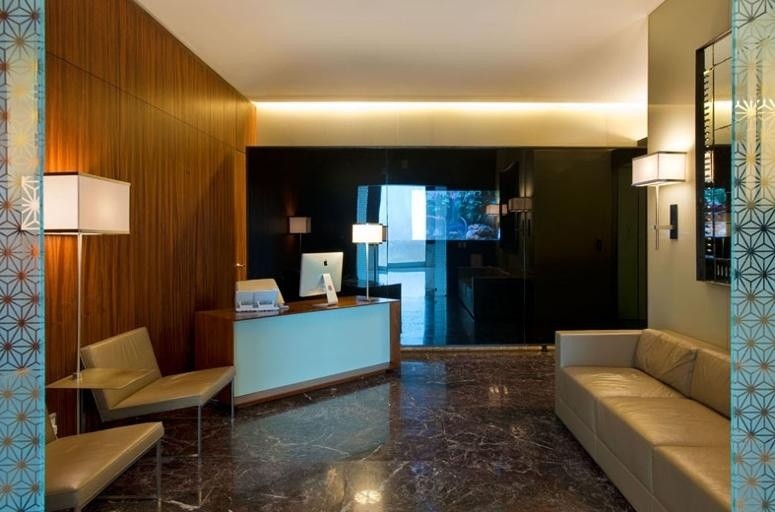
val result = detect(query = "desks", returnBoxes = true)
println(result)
[193,294,400,411]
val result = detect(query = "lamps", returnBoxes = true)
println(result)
[485,204,500,226]
[45,169,133,436]
[507,198,525,232]
[289,216,313,254]
[352,222,384,302]
[630,150,687,249]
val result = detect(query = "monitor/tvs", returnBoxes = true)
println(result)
[298,251,345,307]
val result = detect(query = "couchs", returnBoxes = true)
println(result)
[457,246,534,320]
[551,328,730,512]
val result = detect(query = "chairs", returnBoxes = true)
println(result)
[235,278,286,306]
[47,325,238,511]
